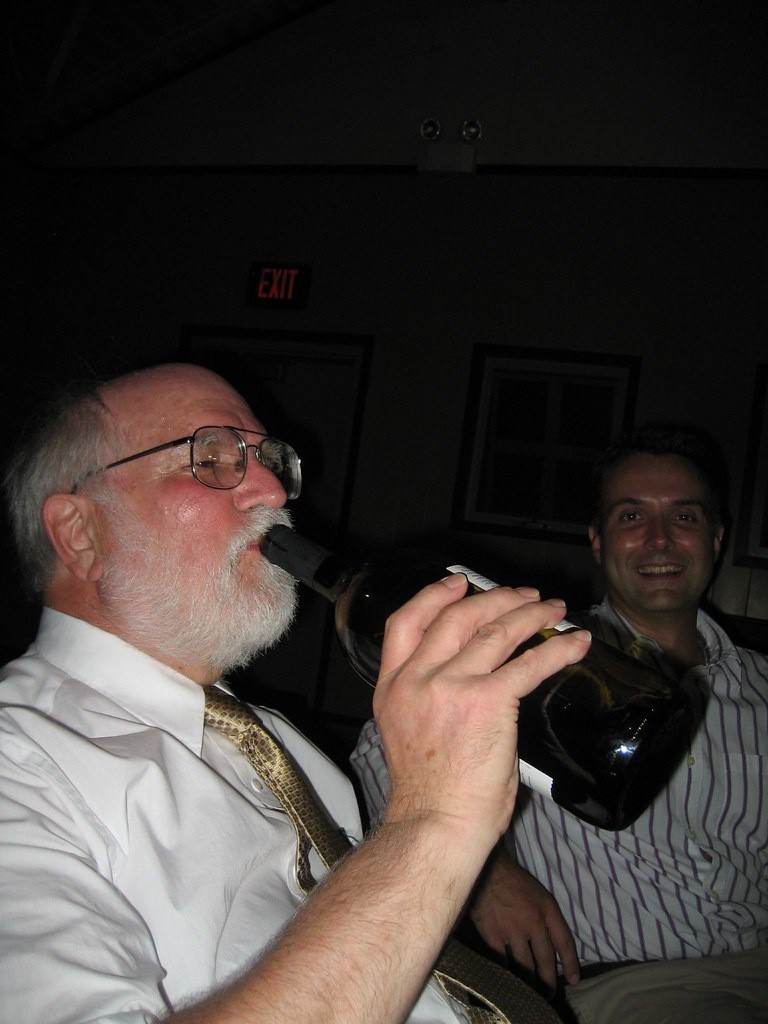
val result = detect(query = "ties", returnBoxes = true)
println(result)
[197,683,563,1024]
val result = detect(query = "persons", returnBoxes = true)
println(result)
[1,362,592,1024]
[351,418,767,1022]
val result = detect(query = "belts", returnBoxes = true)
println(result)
[554,959,642,989]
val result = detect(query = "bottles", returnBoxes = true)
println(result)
[259,524,698,832]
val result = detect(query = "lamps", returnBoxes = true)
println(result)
[416,118,482,171]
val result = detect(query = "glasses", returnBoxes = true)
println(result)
[71,425,303,504]
